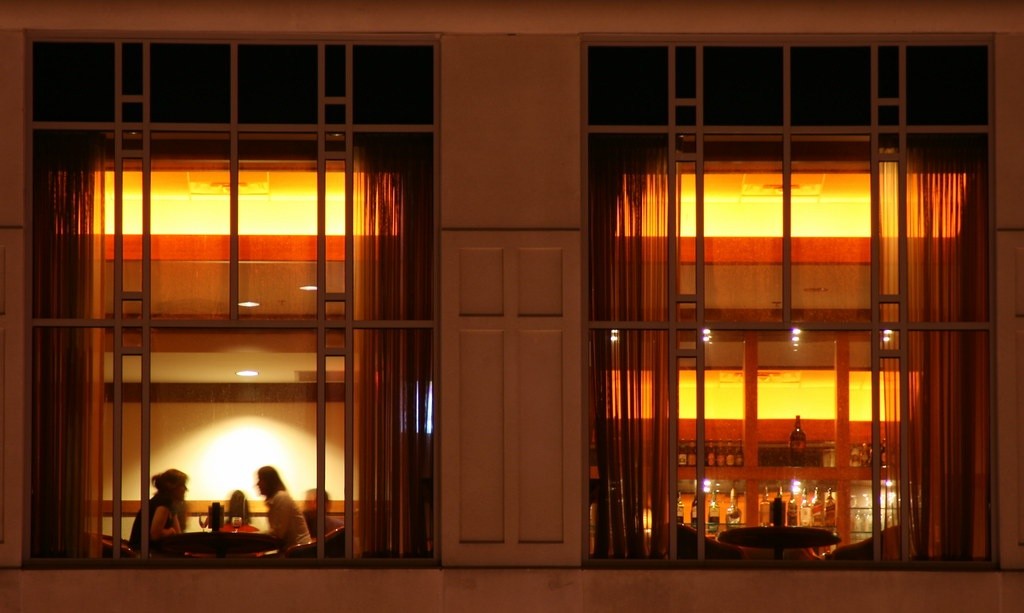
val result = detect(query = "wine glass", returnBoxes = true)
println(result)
[199,513,208,533]
[231,517,242,533]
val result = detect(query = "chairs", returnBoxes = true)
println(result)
[100,533,138,558]
[676,523,747,562]
[830,529,885,561]
[284,524,346,561]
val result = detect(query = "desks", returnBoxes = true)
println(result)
[718,525,842,561]
[150,529,281,563]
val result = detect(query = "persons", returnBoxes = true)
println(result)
[257,465,309,547]
[304,488,345,537]
[223,490,259,533]
[130,468,189,551]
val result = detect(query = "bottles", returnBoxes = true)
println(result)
[677,479,835,534]
[678,439,744,467]
[851,438,886,467]
[789,416,809,466]
